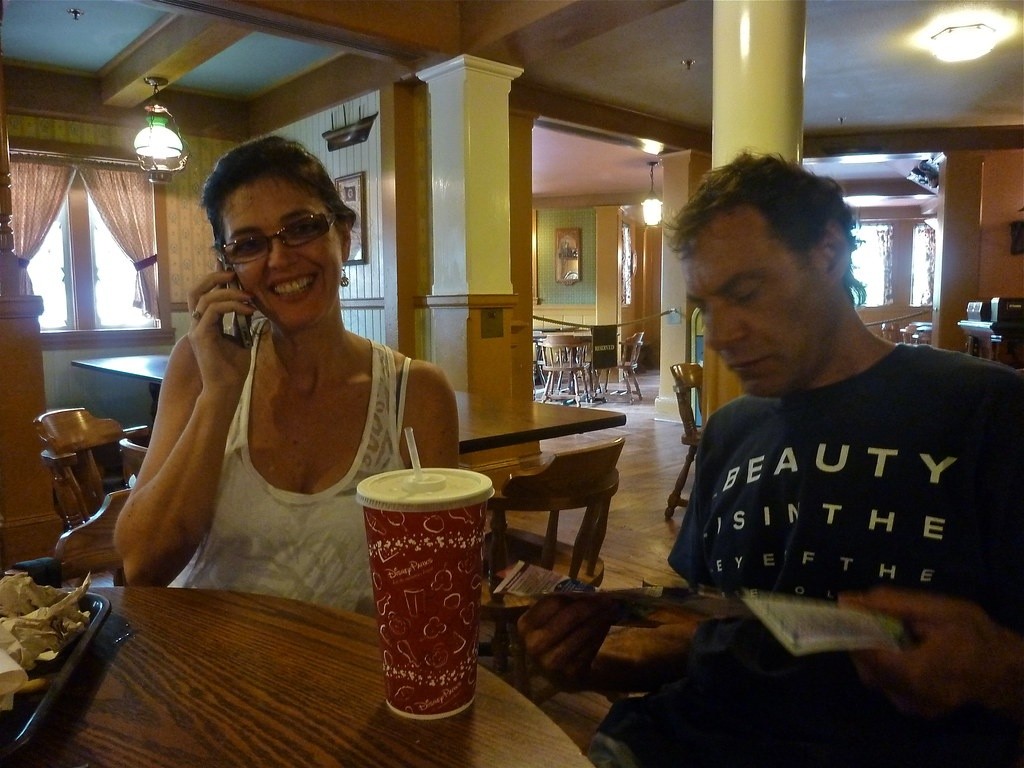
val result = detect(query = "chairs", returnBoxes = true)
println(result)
[665,361,705,521]
[533,332,547,393]
[4,489,135,582]
[539,334,608,411]
[469,432,626,690]
[26,407,151,535]
[605,331,644,403]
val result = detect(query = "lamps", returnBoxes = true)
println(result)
[908,5,1019,61]
[641,160,665,227]
[133,75,191,183]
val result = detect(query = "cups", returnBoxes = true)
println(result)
[355,469,494,721]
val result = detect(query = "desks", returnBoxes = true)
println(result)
[70,352,172,416]
[453,387,629,489]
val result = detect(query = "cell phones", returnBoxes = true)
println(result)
[227,278,254,350]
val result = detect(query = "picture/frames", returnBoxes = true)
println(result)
[335,171,370,266]
[553,227,582,285]
[620,220,636,305]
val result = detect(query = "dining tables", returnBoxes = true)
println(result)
[18,586,594,768]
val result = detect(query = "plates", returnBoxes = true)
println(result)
[0,593,111,768]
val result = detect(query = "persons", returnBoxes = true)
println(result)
[515,152,1024,768]
[113,135,461,618]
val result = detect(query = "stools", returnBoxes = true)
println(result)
[879,323,934,346]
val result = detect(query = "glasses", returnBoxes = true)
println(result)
[222,213,337,264]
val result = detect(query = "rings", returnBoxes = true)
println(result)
[192,309,202,321]
